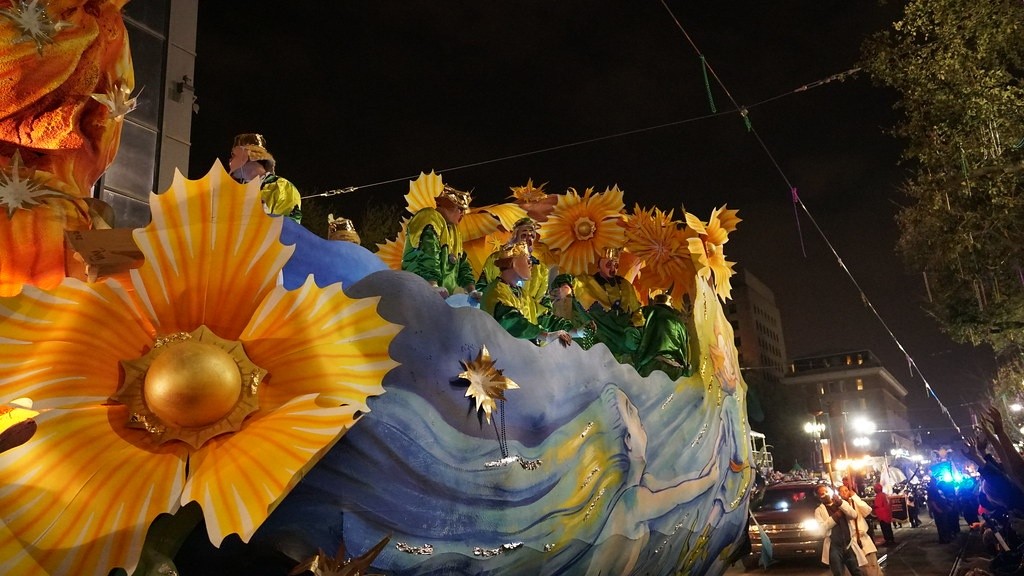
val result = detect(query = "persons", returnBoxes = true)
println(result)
[740,407,1024,576]
[476,217,553,309]
[545,246,691,378]
[400,183,484,303]
[228,133,303,225]
[480,242,573,347]
[327,213,361,245]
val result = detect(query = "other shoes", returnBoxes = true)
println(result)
[916,520,922,527]
[883,540,894,546]
[912,524,917,527]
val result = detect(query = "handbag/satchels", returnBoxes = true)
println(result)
[857,540,863,549]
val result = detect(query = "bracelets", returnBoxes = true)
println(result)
[470,290,477,295]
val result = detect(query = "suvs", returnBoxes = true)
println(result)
[740,479,840,571]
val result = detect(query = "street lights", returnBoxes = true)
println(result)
[805,422,826,473]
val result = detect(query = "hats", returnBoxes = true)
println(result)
[874,483,882,489]
[654,294,674,309]
[328,213,361,244]
[494,241,529,267]
[232,133,275,174]
[600,246,619,258]
[551,273,574,288]
[510,217,537,242]
[435,185,472,208]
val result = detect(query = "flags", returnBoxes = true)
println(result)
[759,526,773,574]
